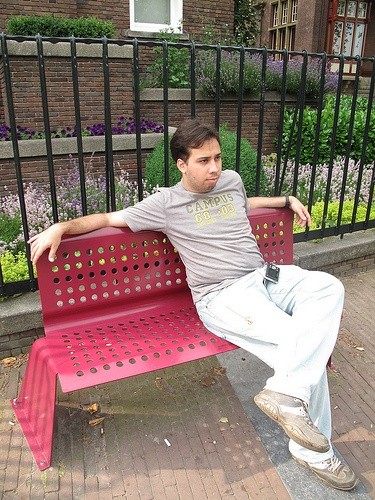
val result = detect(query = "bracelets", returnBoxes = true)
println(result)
[285,196,290,208]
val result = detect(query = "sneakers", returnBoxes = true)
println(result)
[291,446,357,492]
[254,388,331,453]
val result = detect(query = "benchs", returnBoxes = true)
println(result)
[9,204,337,472]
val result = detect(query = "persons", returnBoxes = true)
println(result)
[27,119,359,491]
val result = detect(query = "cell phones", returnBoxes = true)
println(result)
[265,263,280,284]
